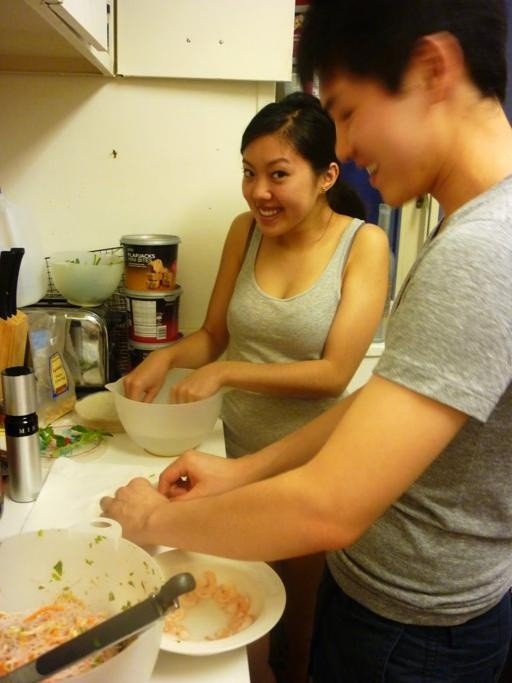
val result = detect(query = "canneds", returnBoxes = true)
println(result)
[120,233,183,370]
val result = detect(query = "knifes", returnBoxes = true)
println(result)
[0,246,25,319]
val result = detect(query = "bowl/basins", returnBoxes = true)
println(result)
[153,548,288,655]
[48,251,123,307]
[0,519,166,683]
[105,368,225,456]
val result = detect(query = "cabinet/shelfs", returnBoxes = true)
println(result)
[0,0,295,83]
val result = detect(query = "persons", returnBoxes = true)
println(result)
[99,2,511,683]
[122,91,390,683]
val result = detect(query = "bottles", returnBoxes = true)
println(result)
[1,367,43,502]
